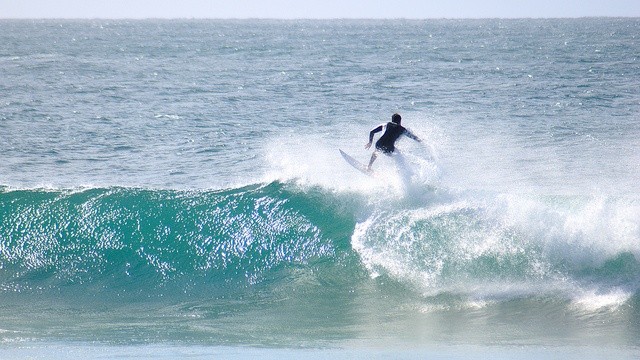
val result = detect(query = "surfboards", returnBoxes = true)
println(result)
[339,149,369,174]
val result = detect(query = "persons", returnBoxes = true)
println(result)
[364,113,422,173]
